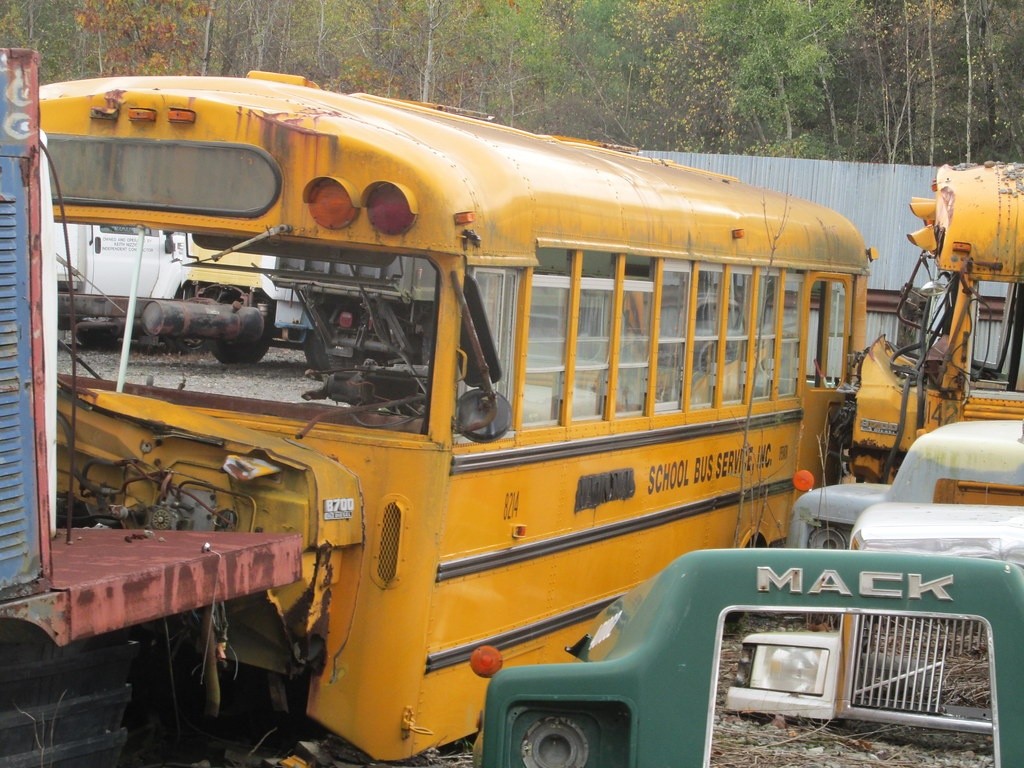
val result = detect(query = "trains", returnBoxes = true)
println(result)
[0,47,1024,768]
